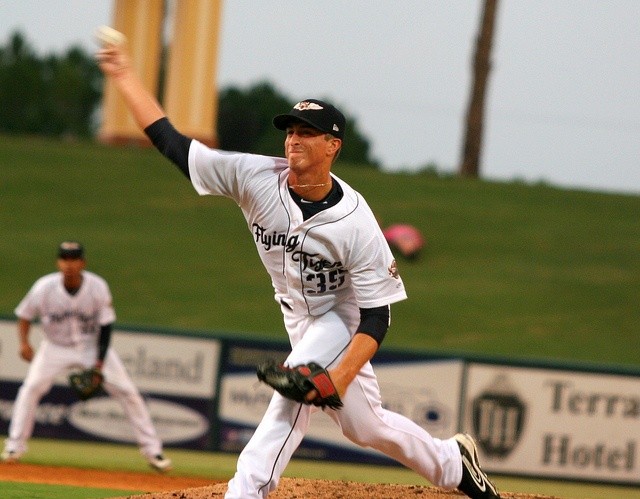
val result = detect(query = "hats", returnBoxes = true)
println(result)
[273,99,345,141]
[58,242,83,257]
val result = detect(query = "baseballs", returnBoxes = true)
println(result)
[97,27,126,50]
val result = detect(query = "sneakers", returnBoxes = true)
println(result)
[148,456,172,470]
[2,452,20,461]
[453,432,501,499]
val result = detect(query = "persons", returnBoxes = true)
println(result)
[1,241,172,471]
[94,43,501,498]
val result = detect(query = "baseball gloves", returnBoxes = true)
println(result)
[256,353,344,411]
[69,366,103,399]
